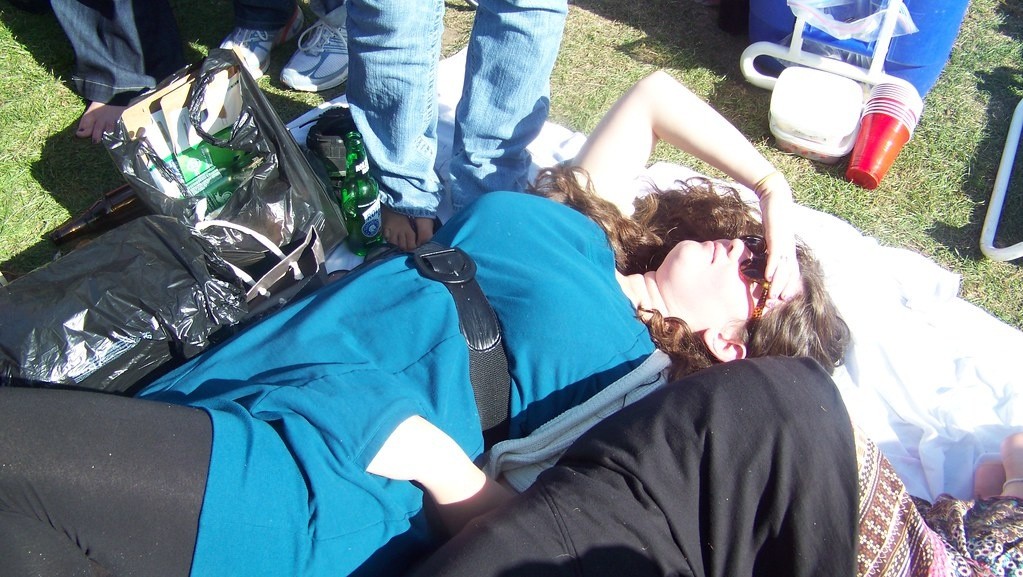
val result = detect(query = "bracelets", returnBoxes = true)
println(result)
[753,171,781,194]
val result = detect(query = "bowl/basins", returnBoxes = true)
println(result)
[769,67,864,164]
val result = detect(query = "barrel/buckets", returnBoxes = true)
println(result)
[750,0,971,96]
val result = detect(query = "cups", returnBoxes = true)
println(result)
[848,82,926,187]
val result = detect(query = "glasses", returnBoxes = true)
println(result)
[738,233,770,348]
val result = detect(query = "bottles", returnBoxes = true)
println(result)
[153,126,267,217]
[343,132,383,255]
[49,182,149,246]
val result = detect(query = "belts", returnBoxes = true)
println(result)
[407,240,510,453]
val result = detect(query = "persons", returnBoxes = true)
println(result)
[414,355,1023,577]
[346,0,567,249]
[0,71,850,577]
[50,0,350,145]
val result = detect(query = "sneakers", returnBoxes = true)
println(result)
[219,3,304,81]
[279,0,350,92]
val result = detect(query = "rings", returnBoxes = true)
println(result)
[780,255,790,260]
